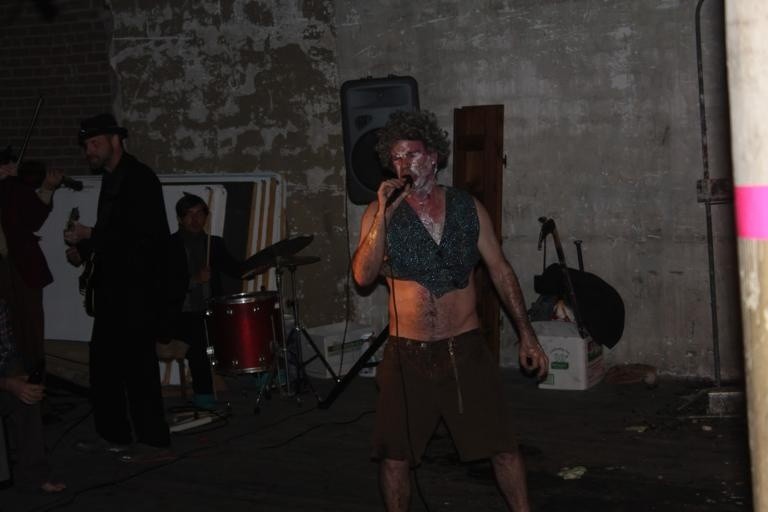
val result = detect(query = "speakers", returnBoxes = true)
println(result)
[340,73,421,206]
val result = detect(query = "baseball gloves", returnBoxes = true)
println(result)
[609,363,658,385]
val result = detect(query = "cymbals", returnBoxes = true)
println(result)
[277,258,320,267]
[247,233,314,267]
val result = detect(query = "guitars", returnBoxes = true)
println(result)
[66,206,100,318]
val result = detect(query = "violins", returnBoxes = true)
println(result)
[0,151,83,190]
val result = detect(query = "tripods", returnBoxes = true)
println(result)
[255,256,390,414]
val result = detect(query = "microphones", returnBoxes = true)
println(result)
[60,175,83,191]
[385,175,413,209]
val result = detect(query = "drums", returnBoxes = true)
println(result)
[207,289,284,377]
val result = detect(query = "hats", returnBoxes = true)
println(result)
[77,112,129,146]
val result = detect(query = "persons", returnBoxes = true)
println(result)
[162,190,249,415]
[1,374,49,406]
[0,144,68,390]
[348,108,551,511]
[65,113,178,466]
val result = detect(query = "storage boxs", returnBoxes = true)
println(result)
[530,317,605,391]
[297,318,376,379]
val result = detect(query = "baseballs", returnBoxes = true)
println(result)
[644,370,656,384]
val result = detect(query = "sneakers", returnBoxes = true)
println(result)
[71,435,177,464]
